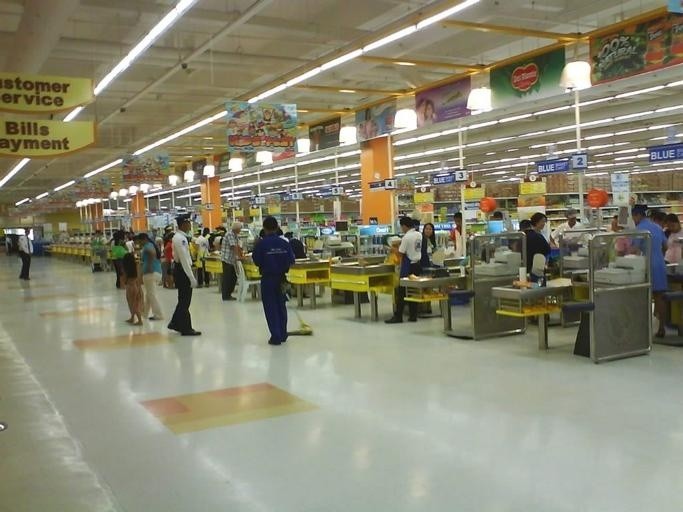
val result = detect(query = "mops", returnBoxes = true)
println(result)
[285,293,313,335]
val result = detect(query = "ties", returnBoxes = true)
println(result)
[27,237,31,254]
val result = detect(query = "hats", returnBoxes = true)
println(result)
[24,226,32,230]
[566,208,578,219]
[175,213,192,224]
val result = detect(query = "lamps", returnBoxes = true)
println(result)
[562,32,591,93]
[0,0,493,208]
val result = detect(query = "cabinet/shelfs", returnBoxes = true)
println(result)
[417,200,464,230]
[629,188,683,230]
[544,190,618,232]
[490,195,519,213]
[272,210,335,234]
[336,208,365,224]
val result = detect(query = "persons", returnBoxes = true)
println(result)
[16,224,36,281]
[249,212,296,346]
[363,108,378,138]
[414,97,438,125]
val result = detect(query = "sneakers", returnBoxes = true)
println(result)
[182,329,202,336]
[149,315,164,321]
[133,321,143,326]
[203,284,209,287]
[268,339,281,345]
[19,276,25,279]
[383,315,404,324]
[125,318,135,323]
[222,295,237,301]
[167,319,182,333]
[24,278,30,280]
[196,285,203,288]
[408,315,417,322]
[277,335,287,343]
[655,328,666,338]
